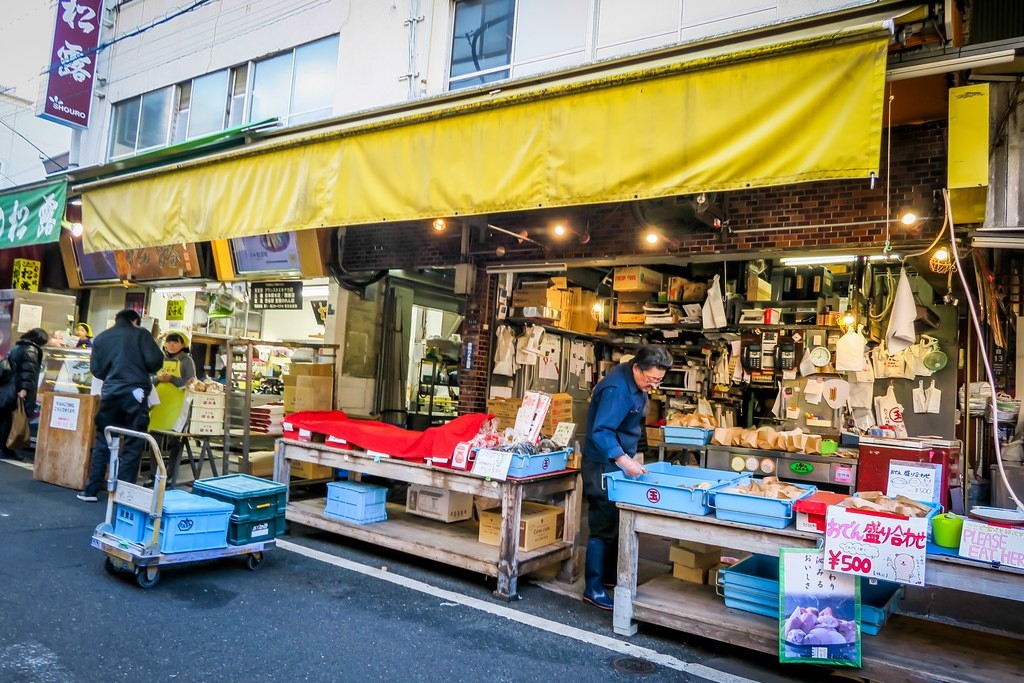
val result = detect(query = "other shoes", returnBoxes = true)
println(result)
[143,475,154,486]
[0,449,23,461]
[77,492,98,501]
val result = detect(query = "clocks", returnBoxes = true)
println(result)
[810,347,832,368]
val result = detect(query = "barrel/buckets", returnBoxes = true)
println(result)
[931,513,963,549]
[820,438,838,456]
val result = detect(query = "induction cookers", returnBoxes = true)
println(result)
[658,369,702,393]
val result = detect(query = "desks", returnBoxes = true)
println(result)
[613,502,1024,683]
[150,429,283,489]
[273,438,584,603]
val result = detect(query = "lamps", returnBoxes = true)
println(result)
[433,218,448,231]
[60,203,83,237]
[780,254,899,267]
[929,247,953,274]
[591,301,603,321]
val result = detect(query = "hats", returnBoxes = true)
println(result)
[74,322,93,338]
[163,330,192,348]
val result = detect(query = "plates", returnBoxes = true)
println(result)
[970,509,1024,526]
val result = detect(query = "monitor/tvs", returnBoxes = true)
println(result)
[70,234,121,283]
[230,229,302,276]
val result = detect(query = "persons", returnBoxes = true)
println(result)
[0,327,49,461]
[76,323,93,347]
[144,330,197,488]
[219,366,234,387]
[77,309,164,502]
[581,344,673,610]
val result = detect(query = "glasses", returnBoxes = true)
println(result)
[637,363,663,385]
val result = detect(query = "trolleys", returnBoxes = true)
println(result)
[91,425,277,588]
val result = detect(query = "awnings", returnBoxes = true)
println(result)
[0,117,278,250]
[73,6,925,255]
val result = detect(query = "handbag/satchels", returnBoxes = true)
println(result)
[6,396,30,450]
[0,358,12,384]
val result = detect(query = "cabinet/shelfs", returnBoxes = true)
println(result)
[406,358,460,431]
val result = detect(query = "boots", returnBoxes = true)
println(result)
[583,538,614,610]
[604,580,617,586]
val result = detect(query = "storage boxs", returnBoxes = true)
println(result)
[601,265,943,635]
[512,276,575,330]
[486,393,573,436]
[406,482,473,522]
[192,473,288,523]
[323,431,360,450]
[468,448,573,479]
[140,497,235,554]
[226,512,286,546]
[323,481,389,525]
[479,501,564,553]
[238,361,335,479]
[114,489,198,543]
[432,445,476,471]
[184,388,225,435]
